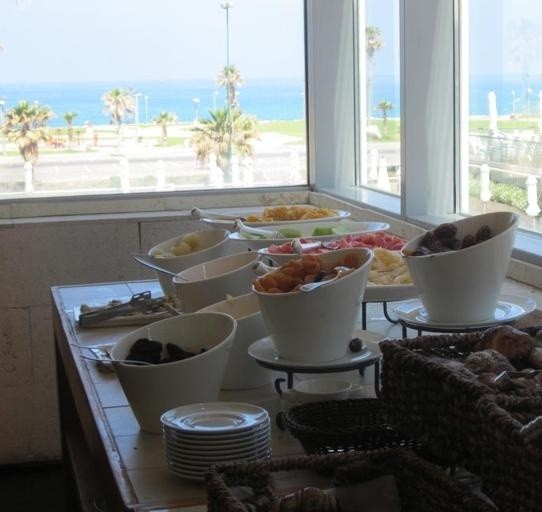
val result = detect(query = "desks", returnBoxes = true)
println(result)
[51,280,419,512]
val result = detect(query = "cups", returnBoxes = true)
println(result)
[278,377,361,403]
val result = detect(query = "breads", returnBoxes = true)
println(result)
[268,485,340,512]
[465,324,542,384]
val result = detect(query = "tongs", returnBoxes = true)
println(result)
[78,291,163,325]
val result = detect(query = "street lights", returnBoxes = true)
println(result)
[212,90,219,111]
[527,88,533,116]
[511,90,517,115]
[193,96,200,118]
[221,0,236,118]
[134,92,142,128]
[144,95,150,126]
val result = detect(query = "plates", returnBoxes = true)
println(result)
[249,233,419,303]
[89,341,116,371]
[393,293,536,329]
[225,221,391,252]
[235,207,354,221]
[247,325,385,373]
[159,400,271,482]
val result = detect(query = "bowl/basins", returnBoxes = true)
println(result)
[251,247,375,365]
[148,227,230,312]
[110,311,238,435]
[193,294,277,395]
[400,210,519,322]
[171,250,261,347]
